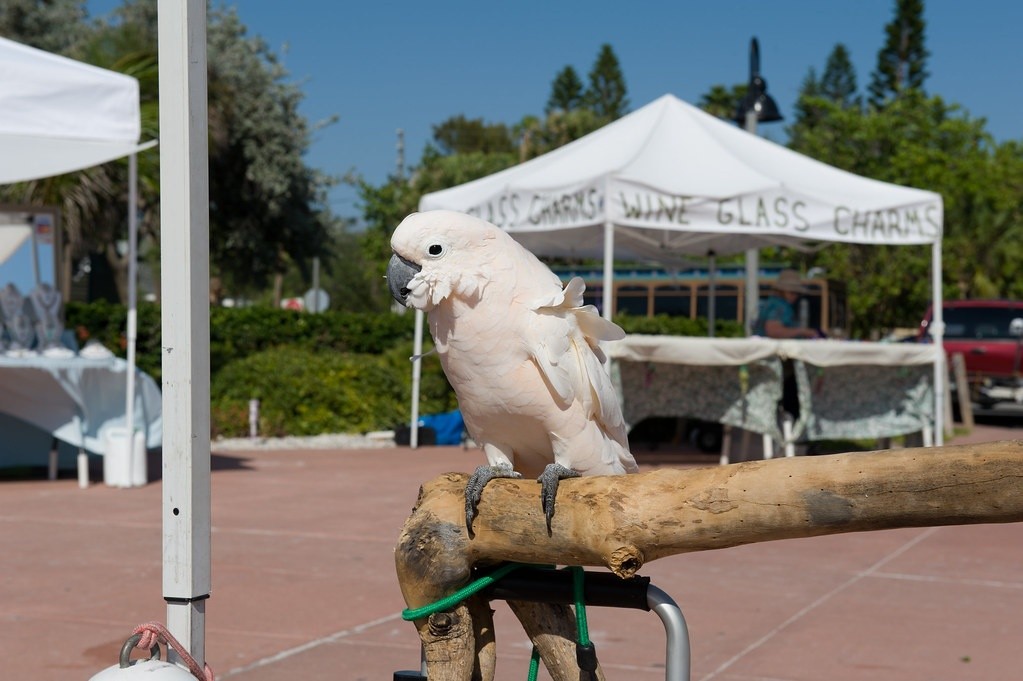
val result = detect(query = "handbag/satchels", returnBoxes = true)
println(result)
[393,409,465,446]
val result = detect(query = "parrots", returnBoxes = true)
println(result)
[387,207,642,541]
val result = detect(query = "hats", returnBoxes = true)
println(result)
[770,270,808,293]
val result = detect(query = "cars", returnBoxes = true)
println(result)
[917,300,1023,380]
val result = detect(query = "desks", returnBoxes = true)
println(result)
[0,355,162,487]
[602,334,945,465]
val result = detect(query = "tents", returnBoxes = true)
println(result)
[0,37,159,487]
[410,93,943,448]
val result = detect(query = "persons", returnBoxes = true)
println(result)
[87,248,121,304]
[756,269,818,337]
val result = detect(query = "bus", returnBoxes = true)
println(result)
[542,263,850,342]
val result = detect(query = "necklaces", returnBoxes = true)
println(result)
[35,290,57,330]
